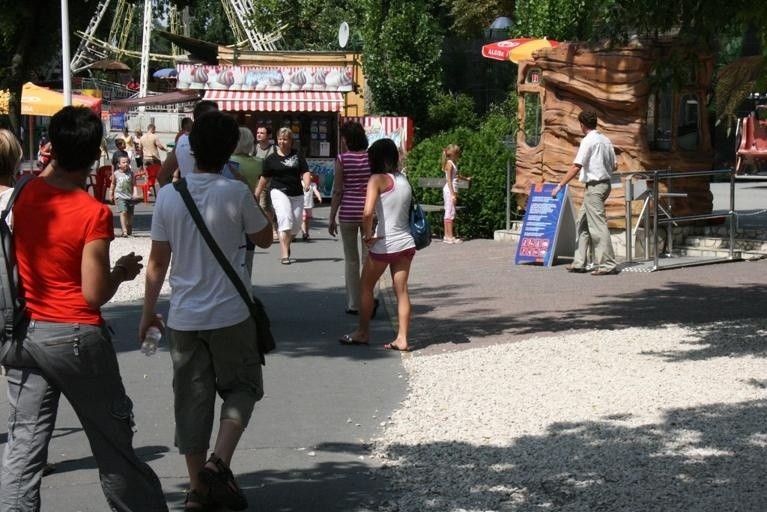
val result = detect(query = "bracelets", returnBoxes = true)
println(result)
[363,234,374,243]
[558,184,563,189]
[114,263,134,281]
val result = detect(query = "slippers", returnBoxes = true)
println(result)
[338,334,368,345]
[383,343,409,351]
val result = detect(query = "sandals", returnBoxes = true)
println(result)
[184,489,211,511]
[200,454,249,511]
[127,225,132,235]
[281,249,290,264]
[122,231,127,237]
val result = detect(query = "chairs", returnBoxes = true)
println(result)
[84,162,160,206]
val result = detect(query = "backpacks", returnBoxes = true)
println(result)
[0,175,35,362]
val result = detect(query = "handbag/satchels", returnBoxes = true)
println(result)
[251,296,276,353]
[410,205,431,249]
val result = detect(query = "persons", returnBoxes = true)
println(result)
[139,111,274,511]
[37,131,53,170]
[0,105,170,512]
[442,144,473,245]
[0,129,55,477]
[223,124,322,265]
[337,138,417,353]
[549,110,621,276]
[94,102,220,236]
[125,75,140,99]
[328,120,381,317]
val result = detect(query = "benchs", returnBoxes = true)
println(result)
[412,176,468,238]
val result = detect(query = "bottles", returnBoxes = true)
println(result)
[139,314,165,356]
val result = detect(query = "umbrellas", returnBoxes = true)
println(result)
[480,36,565,146]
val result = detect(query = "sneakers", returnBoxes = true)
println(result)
[444,236,463,245]
[372,299,378,320]
[345,305,358,313]
[566,265,585,272]
[591,270,621,275]
